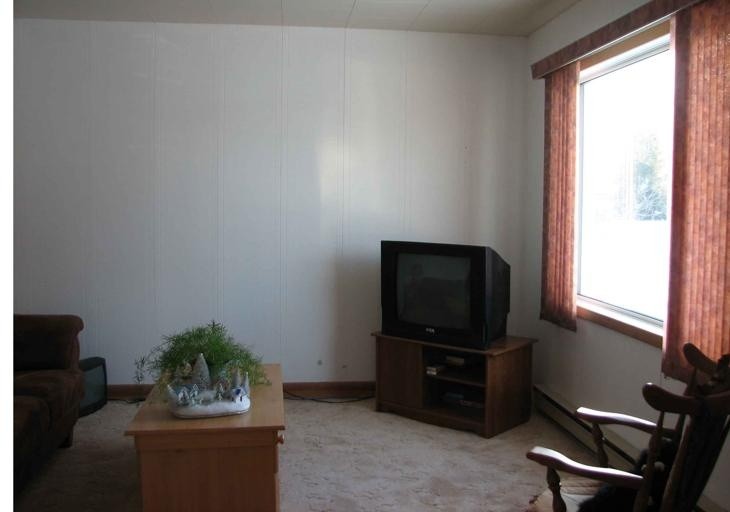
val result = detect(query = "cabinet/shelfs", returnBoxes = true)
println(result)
[371,330,538,439]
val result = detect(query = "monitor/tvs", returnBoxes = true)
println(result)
[379,240,510,351]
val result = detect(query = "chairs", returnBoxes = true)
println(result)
[526,342,730,512]
[14,314,84,496]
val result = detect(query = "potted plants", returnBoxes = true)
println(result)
[132,319,272,391]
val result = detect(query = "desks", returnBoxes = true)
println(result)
[125,363,286,512]
